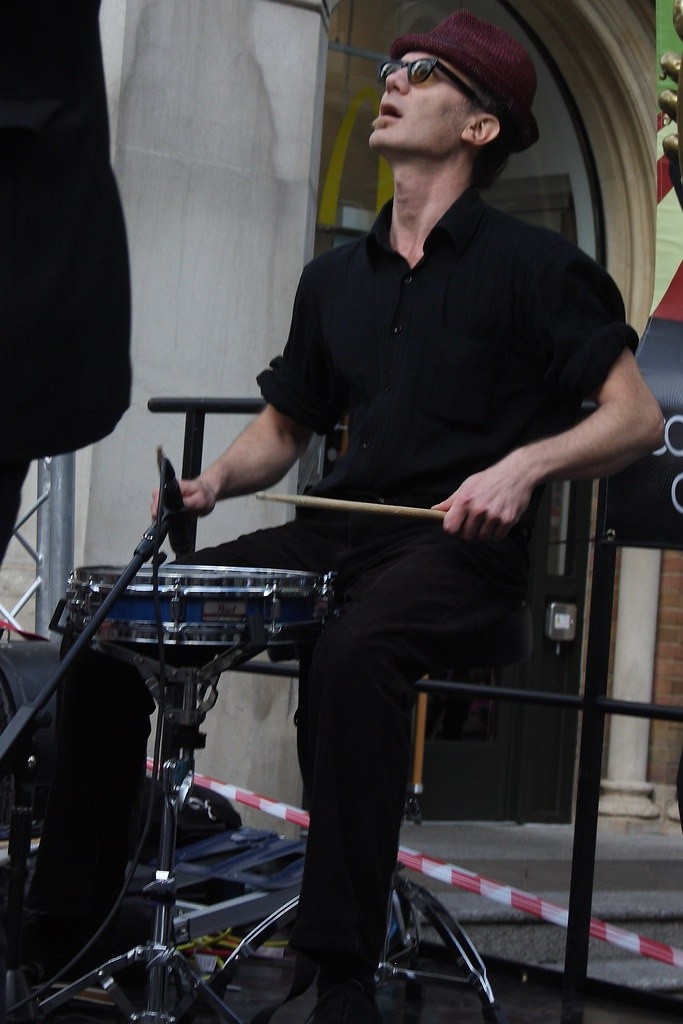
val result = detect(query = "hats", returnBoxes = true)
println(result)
[390,10,550,145]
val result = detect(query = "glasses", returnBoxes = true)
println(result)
[377,55,489,111]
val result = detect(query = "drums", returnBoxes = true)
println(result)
[65,565,338,646]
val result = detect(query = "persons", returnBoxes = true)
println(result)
[20,13,666,1024]
[0,0,133,562]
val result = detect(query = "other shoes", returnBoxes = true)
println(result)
[305,979,381,1023]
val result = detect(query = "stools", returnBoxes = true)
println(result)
[212,605,534,1019]
